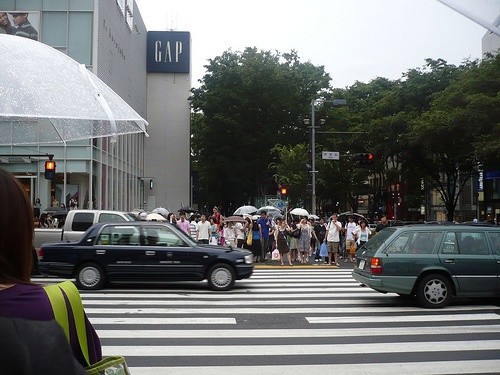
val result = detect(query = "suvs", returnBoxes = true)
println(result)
[351,221,500,310]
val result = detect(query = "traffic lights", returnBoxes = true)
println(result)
[353,153,374,162]
[281,184,288,200]
[44,161,56,179]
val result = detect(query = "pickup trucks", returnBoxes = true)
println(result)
[33,208,135,252]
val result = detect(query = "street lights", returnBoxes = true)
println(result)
[304,98,349,216]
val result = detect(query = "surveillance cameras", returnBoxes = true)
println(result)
[320,118,326,126]
[303,119,310,125]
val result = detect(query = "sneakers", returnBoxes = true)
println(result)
[349,258,354,263]
[288,262,293,266]
[332,261,335,264]
[339,256,343,260]
[353,258,357,262]
[344,258,349,263]
[281,262,285,266]
[324,261,328,264]
[334,262,341,266]
[328,263,332,266]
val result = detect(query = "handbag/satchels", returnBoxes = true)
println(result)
[215,229,220,240]
[319,243,329,257]
[84,356,131,374]
[247,228,253,245]
[293,228,301,238]
[271,249,280,261]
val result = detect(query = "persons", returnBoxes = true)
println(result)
[258,211,271,262]
[118,229,138,245]
[225,221,238,250]
[147,230,176,246]
[167,213,176,224]
[0,167,105,375]
[298,218,312,264]
[52,198,59,207]
[74,199,78,210]
[197,215,212,244]
[212,206,225,246]
[234,215,248,248]
[9,13,38,41]
[33,198,41,219]
[375,214,391,234]
[323,213,372,266]
[0,12,16,35]
[68,197,74,210]
[61,203,65,210]
[189,214,198,241]
[93,199,97,209]
[151,219,157,222]
[269,214,300,266]
[209,216,217,245]
[156,213,162,216]
[250,215,262,263]
[185,213,191,220]
[313,218,326,262]
[177,211,191,237]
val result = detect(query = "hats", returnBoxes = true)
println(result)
[251,216,258,221]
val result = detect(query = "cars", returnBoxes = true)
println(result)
[35,220,255,291]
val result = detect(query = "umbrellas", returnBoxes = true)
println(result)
[309,214,319,219]
[146,213,167,221]
[233,205,257,215]
[257,206,284,225]
[290,208,308,219]
[0,34,150,146]
[151,206,169,215]
[177,207,197,215]
[131,208,147,220]
[225,216,246,222]
